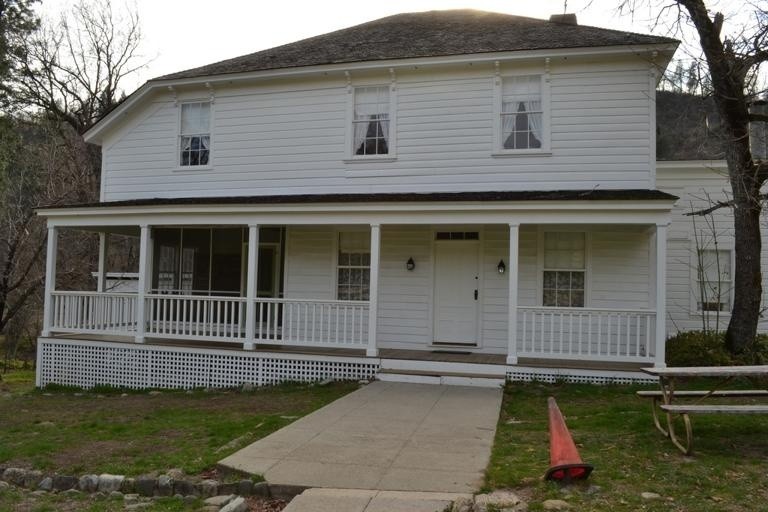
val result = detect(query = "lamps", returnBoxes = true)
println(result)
[406,256,415,270]
[497,259,505,274]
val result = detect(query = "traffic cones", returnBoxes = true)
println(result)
[541,395,594,481]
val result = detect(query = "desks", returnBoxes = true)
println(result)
[640,365,768,455]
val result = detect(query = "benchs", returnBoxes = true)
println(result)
[638,390,768,436]
[660,404,768,457]
